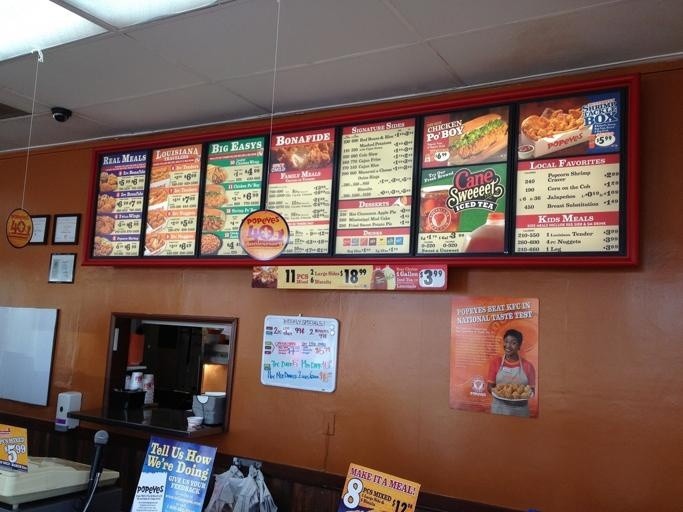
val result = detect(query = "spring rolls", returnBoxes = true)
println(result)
[206,163,226,184]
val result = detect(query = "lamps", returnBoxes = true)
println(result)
[50,107,72,122]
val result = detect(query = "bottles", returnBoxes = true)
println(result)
[464,213,505,252]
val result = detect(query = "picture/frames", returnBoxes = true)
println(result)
[52,213,80,245]
[27,215,50,245]
[47,253,77,283]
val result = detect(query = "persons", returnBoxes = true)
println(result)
[485,329,535,417]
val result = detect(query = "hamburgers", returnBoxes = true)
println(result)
[448,112,510,165]
[205,184,227,208]
[203,209,226,232]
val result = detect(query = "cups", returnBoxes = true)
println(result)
[125,372,154,405]
[420,186,460,233]
[187,417,203,431]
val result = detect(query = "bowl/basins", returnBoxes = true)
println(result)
[518,145,534,160]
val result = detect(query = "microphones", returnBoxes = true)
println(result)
[83,427,110,499]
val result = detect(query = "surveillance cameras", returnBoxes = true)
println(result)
[49,106,73,124]
